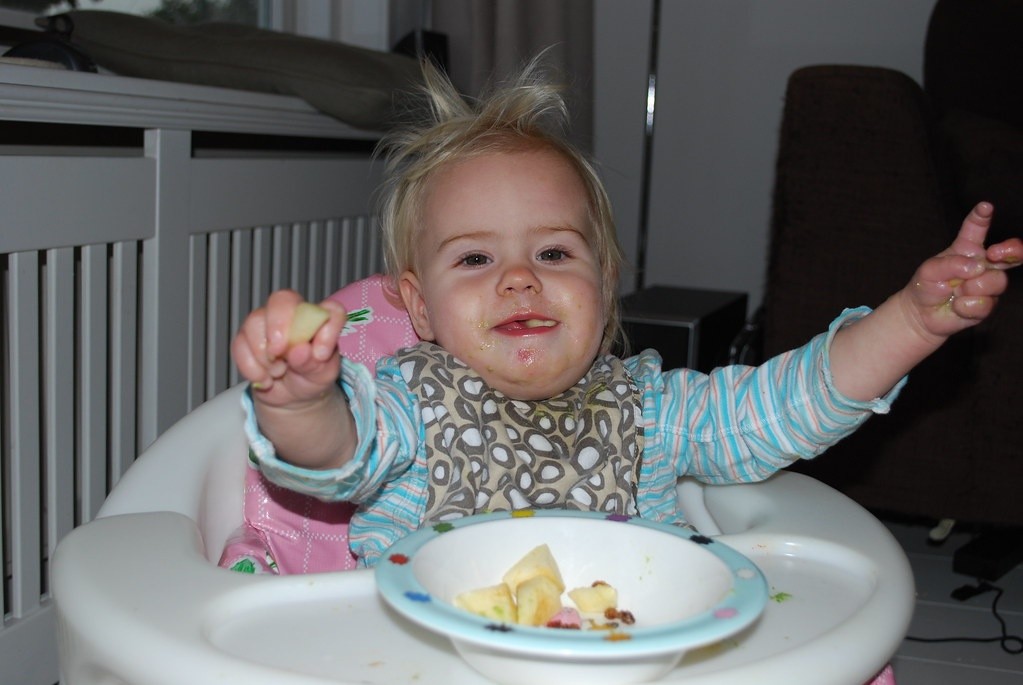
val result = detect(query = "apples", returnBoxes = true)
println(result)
[453,541,617,627]
[278,300,330,359]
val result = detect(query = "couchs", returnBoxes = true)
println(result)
[759,1,1023,534]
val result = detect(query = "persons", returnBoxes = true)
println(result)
[232,71,1023,572]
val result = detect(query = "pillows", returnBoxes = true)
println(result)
[36,10,439,130]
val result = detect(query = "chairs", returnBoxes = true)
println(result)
[50,382,920,685]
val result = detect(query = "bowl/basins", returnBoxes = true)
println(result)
[372,509,769,684]
